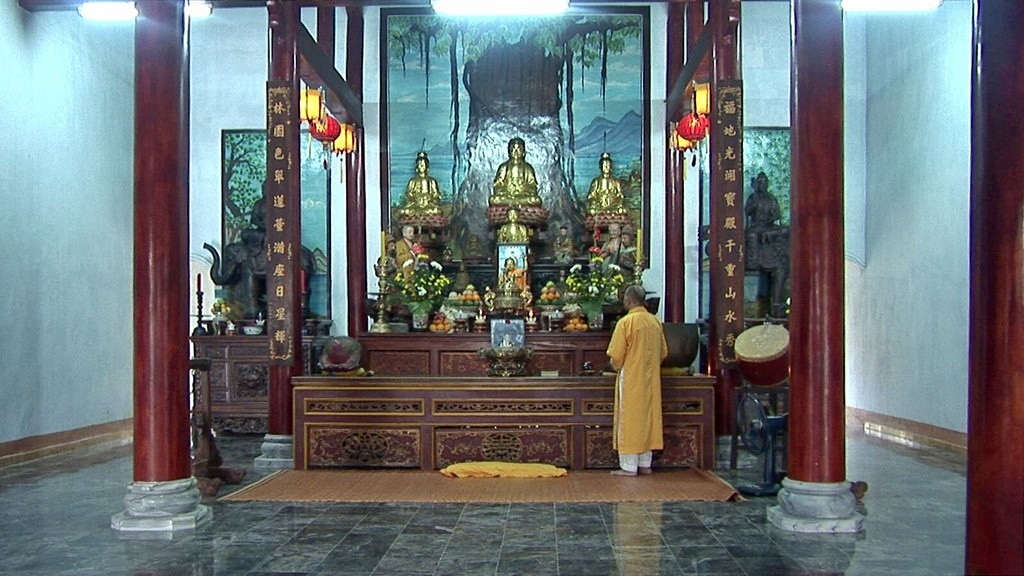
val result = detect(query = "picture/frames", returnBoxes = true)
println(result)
[496,243,528,278]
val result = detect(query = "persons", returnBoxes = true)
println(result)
[243,179,266,243]
[587,153,624,209]
[503,258,527,292]
[491,138,538,196]
[601,223,639,282]
[499,206,528,243]
[402,152,442,206]
[606,285,667,472]
[554,226,574,263]
[464,233,484,258]
[745,172,789,235]
[377,224,422,296]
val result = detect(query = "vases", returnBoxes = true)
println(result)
[587,297,603,329]
[408,301,432,329]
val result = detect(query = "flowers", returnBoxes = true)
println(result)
[566,228,624,297]
[394,243,450,301]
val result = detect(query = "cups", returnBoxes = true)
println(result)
[538,316,549,332]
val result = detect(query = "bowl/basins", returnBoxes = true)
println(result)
[243,326,263,336]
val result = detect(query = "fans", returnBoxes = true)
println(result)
[737,396,787,497]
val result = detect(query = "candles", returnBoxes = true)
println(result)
[301,270,305,292]
[637,229,642,263]
[382,231,385,266]
[197,273,202,291]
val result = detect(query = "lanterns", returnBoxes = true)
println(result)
[330,123,356,183]
[690,79,710,154]
[310,112,342,150]
[674,110,706,157]
[300,81,326,158]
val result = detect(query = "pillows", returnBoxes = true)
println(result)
[439,462,568,478]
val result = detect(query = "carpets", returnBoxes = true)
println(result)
[214,467,749,502]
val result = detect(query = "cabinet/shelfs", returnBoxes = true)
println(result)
[355,332,612,377]
[187,335,335,433]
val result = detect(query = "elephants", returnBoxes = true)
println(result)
[706,227,790,318]
[203,243,317,318]
